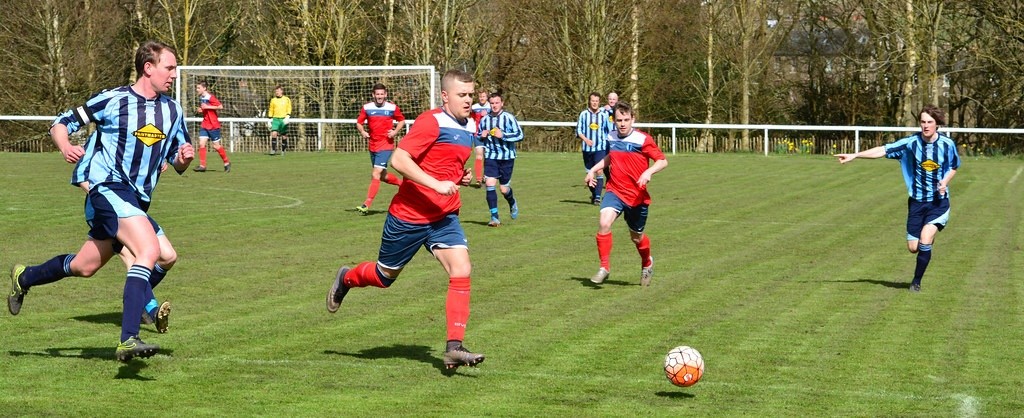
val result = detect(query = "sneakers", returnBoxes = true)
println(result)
[357,205,369,212]
[8,264,32,315]
[224,162,231,173]
[591,267,610,283]
[154,301,172,334]
[193,166,206,172]
[641,256,654,285]
[327,265,351,313]
[592,195,600,205]
[510,198,518,219]
[116,334,160,361]
[487,213,500,228]
[910,280,919,292]
[444,346,485,370]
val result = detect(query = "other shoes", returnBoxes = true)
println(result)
[475,181,481,187]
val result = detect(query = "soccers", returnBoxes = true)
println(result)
[664,345,704,387]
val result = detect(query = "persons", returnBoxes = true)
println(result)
[833,103,960,292]
[355,83,405,213]
[585,101,668,288]
[9,41,196,362]
[471,87,492,187]
[269,86,292,156]
[326,68,485,370]
[478,93,524,226]
[192,81,231,173]
[577,92,619,205]
